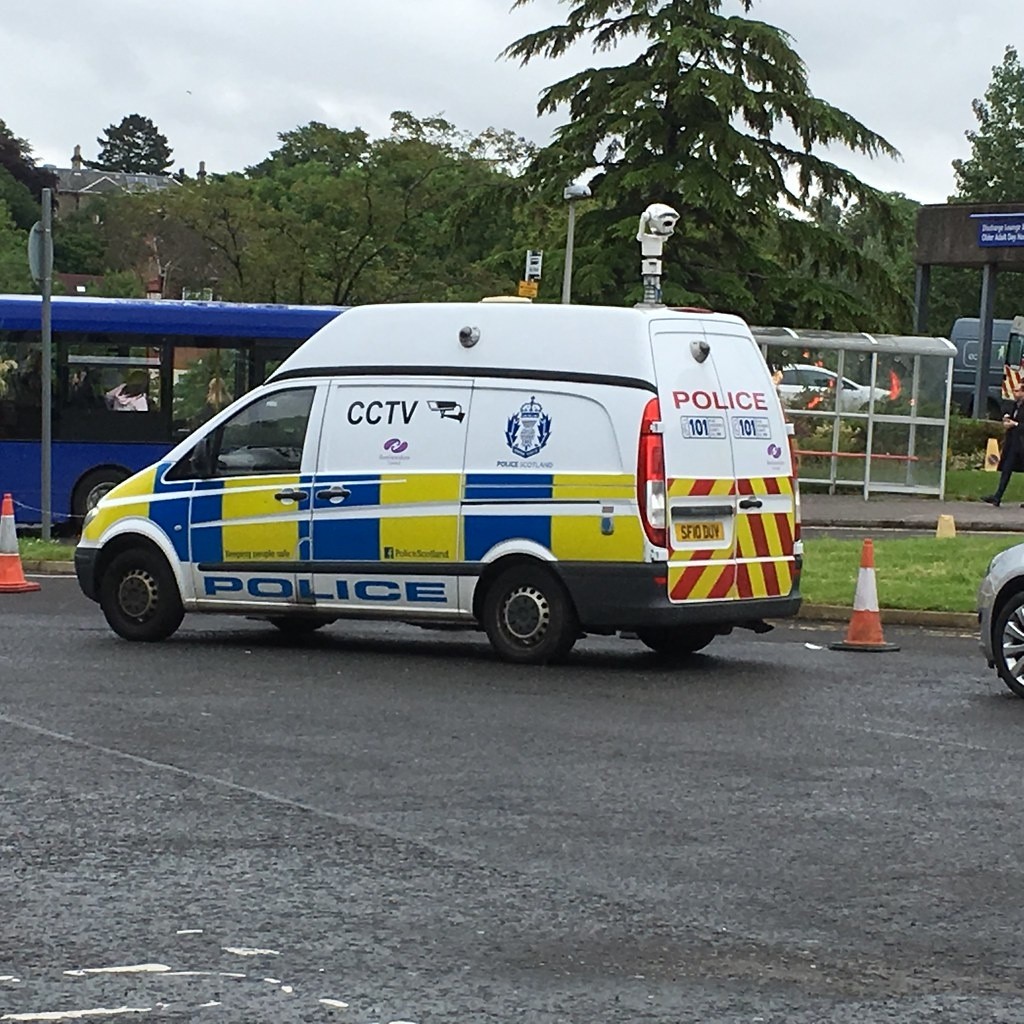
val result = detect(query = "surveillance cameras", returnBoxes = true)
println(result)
[646,204,679,233]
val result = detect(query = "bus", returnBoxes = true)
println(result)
[0,292,350,538]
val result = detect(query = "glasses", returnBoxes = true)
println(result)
[1012,390,1022,392]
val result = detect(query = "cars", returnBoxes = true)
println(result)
[975,543,1024,700]
[766,364,894,421]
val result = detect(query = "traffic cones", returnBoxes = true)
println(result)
[0,493,46,592]
[826,538,902,653]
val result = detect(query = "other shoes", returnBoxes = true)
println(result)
[980,495,1001,507]
[1019,504,1024,508]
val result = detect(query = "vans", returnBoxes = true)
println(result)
[73,300,810,666]
[948,316,1015,421]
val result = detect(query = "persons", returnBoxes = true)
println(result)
[204,377,234,424]
[855,352,879,390]
[104,370,152,414]
[796,349,834,390]
[0,349,104,431]
[769,363,784,403]
[888,366,902,400]
[980,383,1024,511]
